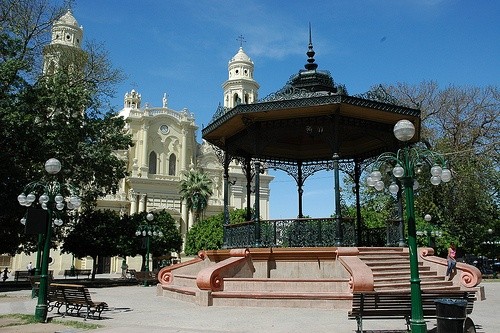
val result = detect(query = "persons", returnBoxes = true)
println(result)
[445,242,457,281]
[463,253,499,279]
[69,265,75,277]
[0,267,11,282]
[26,261,33,282]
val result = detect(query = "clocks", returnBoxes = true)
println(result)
[159,124,170,135]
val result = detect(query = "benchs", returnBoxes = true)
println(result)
[348,290,478,333]
[14,270,54,282]
[129,269,159,286]
[33,282,108,321]
[64,269,92,280]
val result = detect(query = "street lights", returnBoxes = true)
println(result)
[17,158,82,324]
[366,119,452,333]
[136,213,163,287]
[488,229,497,279]
[424,214,432,248]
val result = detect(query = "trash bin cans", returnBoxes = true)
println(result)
[434,295,467,333]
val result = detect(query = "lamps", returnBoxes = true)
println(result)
[228,177,237,186]
[250,185,256,193]
